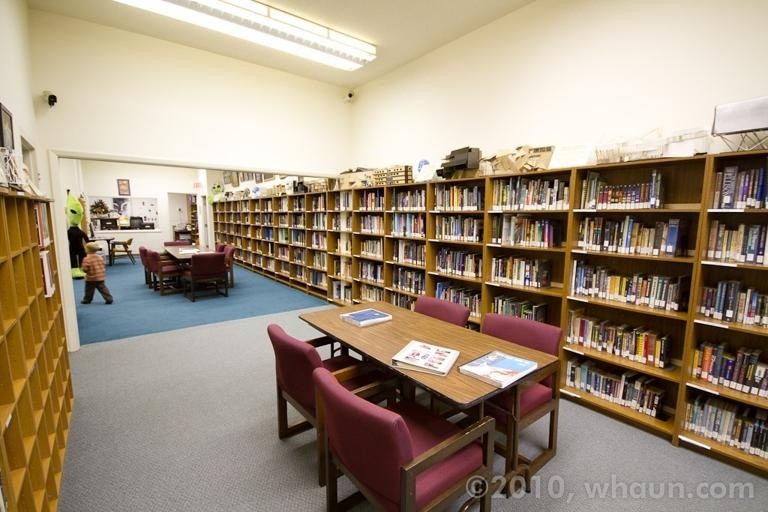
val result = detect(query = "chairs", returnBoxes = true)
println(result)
[183,252,228,302]
[139,247,170,288]
[164,241,190,246]
[111,238,136,266]
[314,365,496,511]
[148,250,186,296]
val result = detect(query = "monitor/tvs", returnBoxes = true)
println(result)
[101,218,117,229]
[139,222,154,229]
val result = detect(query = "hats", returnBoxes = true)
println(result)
[86,243,103,253]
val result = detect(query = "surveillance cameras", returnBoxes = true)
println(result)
[42,89,57,106]
[346,91,354,100]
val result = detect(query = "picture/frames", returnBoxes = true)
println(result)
[0,103,14,154]
[117,179,131,196]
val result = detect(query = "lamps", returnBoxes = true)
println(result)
[111,1,378,74]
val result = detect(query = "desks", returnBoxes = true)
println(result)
[164,246,216,282]
[89,237,115,267]
[164,246,211,260]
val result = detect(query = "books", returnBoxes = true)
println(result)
[681,165,768,462]
[390,189,427,311]
[565,172,680,418]
[491,177,569,324]
[457,350,537,388]
[216,188,385,305]
[391,339,460,377]
[339,308,393,327]
[434,183,484,318]
[34,202,56,298]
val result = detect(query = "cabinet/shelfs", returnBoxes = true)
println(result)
[1,189,75,512]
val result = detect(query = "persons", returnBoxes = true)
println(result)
[64,189,90,280]
[81,242,114,304]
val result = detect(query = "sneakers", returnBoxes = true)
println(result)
[81,300,92,304]
[106,299,113,304]
